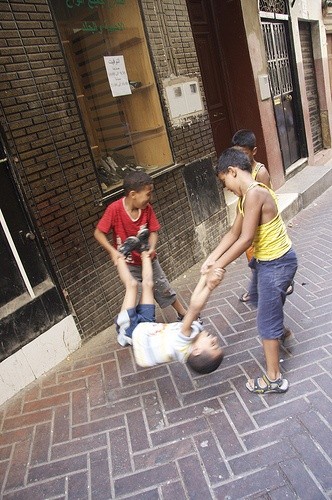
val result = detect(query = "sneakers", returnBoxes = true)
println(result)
[120,235,140,257]
[136,230,151,254]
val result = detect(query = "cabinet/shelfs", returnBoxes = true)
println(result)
[61,40,98,150]
[108,37,162,136]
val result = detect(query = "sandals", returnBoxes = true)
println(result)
[246,375,288,394]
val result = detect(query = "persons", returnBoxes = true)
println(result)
[200,148,298,394]
[94,172,203,325]
[115,228,225,376]
[232,129,294,303]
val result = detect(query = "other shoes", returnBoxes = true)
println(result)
[177,312,202,325]
[286,280,294,295]
[240,291,250,302]
